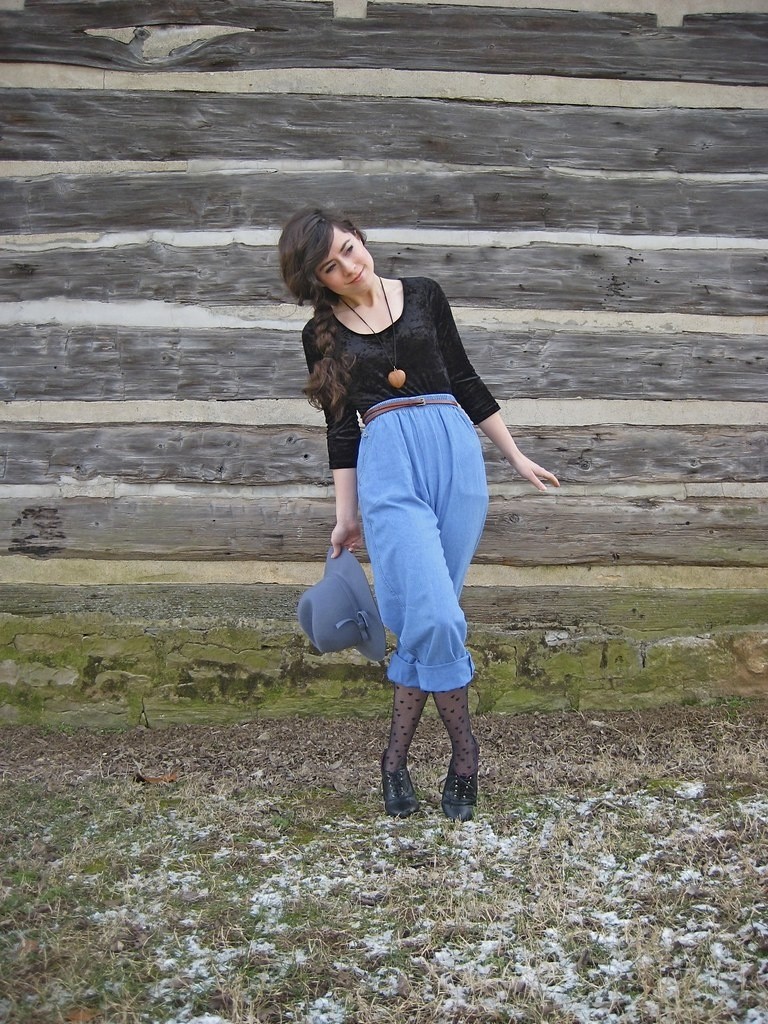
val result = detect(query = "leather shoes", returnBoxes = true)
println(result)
[381,749,419,818]
[442,735,479,822]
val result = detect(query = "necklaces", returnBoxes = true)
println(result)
[337,280,409,390]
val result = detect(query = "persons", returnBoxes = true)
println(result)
[272,203,563,822]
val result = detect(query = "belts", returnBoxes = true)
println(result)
[363,398,457,425]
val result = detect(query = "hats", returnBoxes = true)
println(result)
[298,545,386,663]
